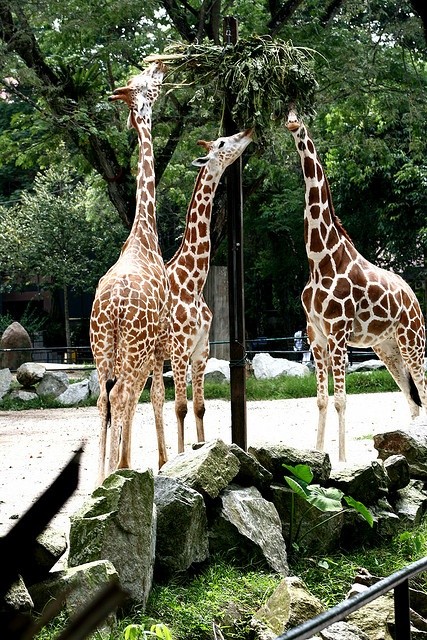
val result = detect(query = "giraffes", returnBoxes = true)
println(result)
[166,130,254,455]
[284,109,427,463]
[90,61,171,486]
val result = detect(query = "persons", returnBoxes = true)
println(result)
[293,329,302,363]
[301,330,312,364]
